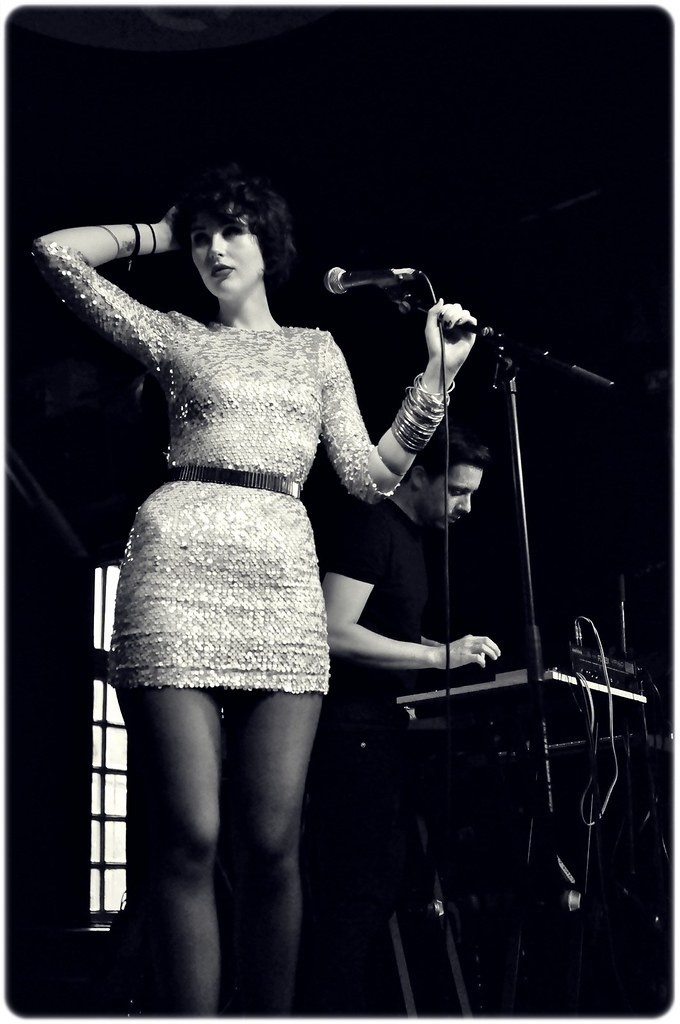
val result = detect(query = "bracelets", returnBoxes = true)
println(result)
[128,222,144,271]
[98,226,120,261]
[144,223,156,257]
[391,372,455,454]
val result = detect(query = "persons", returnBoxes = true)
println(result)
[31,178,483,1016]
[315,421,502,1016]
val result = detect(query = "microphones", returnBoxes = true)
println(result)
[323,266,423,294]
